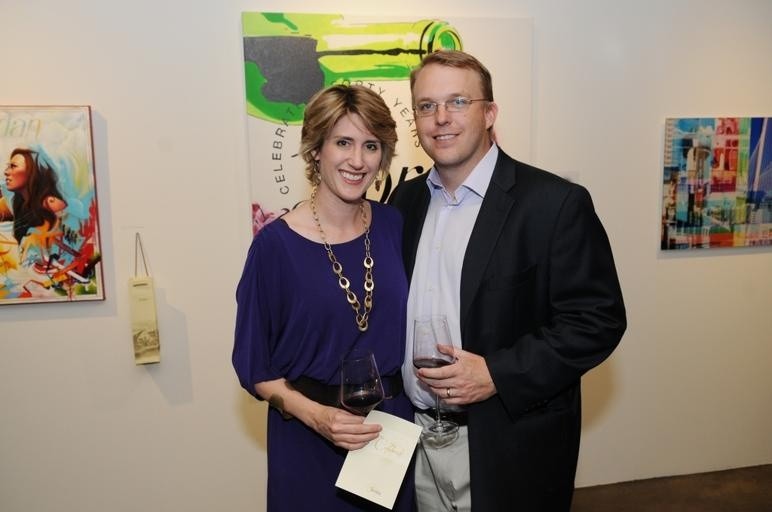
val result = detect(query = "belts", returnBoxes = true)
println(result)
[414,407,464,428]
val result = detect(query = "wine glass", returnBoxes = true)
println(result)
[412,314,459,437]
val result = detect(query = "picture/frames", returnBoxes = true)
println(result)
[0,105,106,306]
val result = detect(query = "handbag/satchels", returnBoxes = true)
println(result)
[126,234,160,365]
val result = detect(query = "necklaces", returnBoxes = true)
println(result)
[310,188,375,333]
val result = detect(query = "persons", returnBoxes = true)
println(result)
[386,49,627,512]
[0,148,67,245]
[232,84,415,512]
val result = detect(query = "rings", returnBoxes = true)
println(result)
[447,389,451,398]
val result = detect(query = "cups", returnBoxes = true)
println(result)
[340,352,385,418]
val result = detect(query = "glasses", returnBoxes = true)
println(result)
[413,96,488,117]
